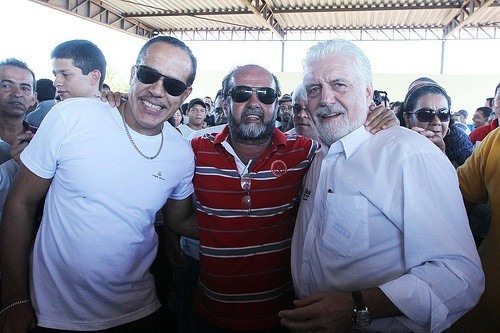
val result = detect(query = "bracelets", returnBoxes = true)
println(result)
[0,299,30,315]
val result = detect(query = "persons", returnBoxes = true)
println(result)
[274,84,321,142]
[149,89,228,333]
[0,40,128,238]
[372,77,500,333]
[0,36,199,333]
[278,39,484,333]
[101,65,400,333]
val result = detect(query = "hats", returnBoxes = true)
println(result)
[278,96,292,105]
[459,110,468,118]
[404,83,445,109]
[187,98,206,111]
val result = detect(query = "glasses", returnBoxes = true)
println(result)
[241,174,251,213]
[409,108,451,122]
[136,65,187,97]
[292,104,310,114]
[223,85,279,105]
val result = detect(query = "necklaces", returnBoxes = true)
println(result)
[123,102,163,159]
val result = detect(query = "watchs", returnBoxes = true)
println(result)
[352,291,372,326]
